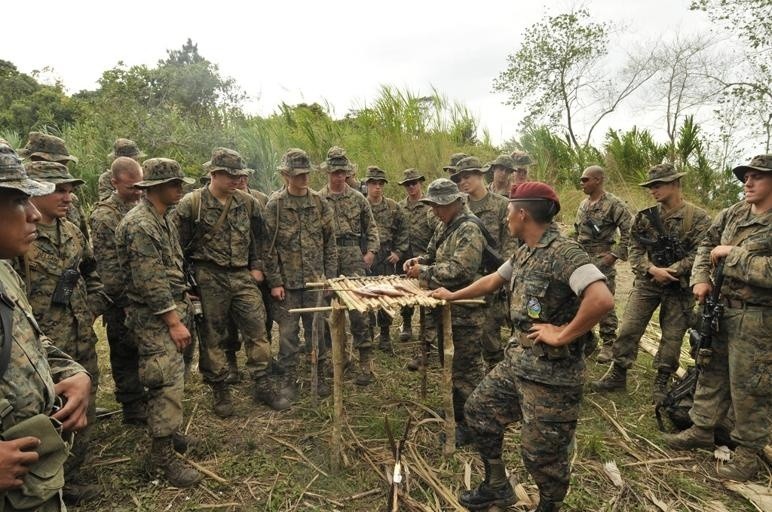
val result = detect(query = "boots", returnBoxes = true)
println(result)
[534,498,561,512]
[253,378,291,411]
[143,437,203,485]
[173,429,205,458]
[399,318,416,341]
[328,350,349,378]
[664,422,714,451]
[406,340,433,371]
[309,361,332,396]
[121,401,152,427]
[212,382,235,419]
[458,459,520,511]
[717,441,762,481]
[224,353,244,385]
[596,340,617,364]
[269,354,281,375]
[650,370,672,407]
[582,363,628,395]
[281,372,302,402]
[379,327,394,354]
[440,422,472,449]
[355,348,375,385]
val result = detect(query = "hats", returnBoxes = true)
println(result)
[638,164,688,189]
[398,168,426,185]
[732,154,771,184]
[346,160,358,179]
[442,153,471,174]
[490,155,518,173]
[133,157,196,190]
[23,161,86,189]
[482,161,495,173]
[418,177,470,207]
[321,146,352,174]
[200,146,250,178]
[508,181,560,216]
[279,148,315,178]
[449,156,489,184]
[510,151,536,171]
[107,138,149,162]
[16,132,80,166]
[360,165,389,184]
[275,147,291,176]
[238,157,255,178]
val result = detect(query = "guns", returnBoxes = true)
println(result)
[182,261,208,353]
[482,240,506,300]
[631,205,689,329]
[669,258,726,402]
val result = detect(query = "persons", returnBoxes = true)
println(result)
[0,129,631,512]
[587,162,713,399]
[655,155,771,480]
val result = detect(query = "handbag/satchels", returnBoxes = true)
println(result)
[481,234,508,273]
[0,413,71,512]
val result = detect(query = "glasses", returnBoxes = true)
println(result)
[578,176,597,183]
[404,181,420,187]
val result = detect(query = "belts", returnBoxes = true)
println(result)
[132,291,187,309]
[580,245,611,255]
[193,260,246,273]
[721,296,770,310]
[335,239,360,247]
[512,327,581,351]
[0,144,57,197]
